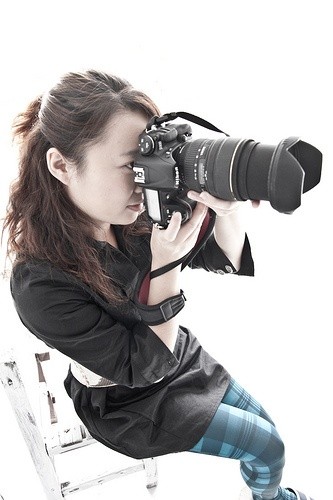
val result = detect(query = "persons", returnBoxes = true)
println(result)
[1,69,314,500]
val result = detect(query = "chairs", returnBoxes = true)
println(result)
[0,340,159,500]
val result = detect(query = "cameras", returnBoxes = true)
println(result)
[135,124,324,231]
